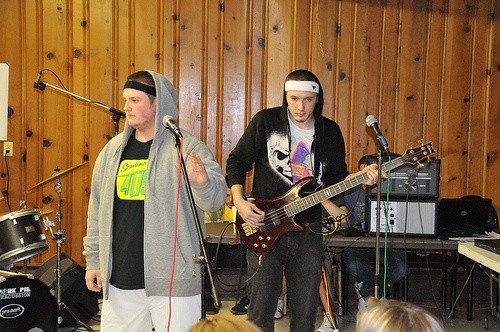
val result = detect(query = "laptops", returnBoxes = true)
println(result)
[437,198,492,239]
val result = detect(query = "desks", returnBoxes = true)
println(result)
[205,223,458,316]
[458,240,500,321]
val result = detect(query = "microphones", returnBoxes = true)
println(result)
[162,115,183,137]
[33,71,42,88]
[366,114,391,157]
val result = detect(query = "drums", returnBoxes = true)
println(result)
[0,208,49,266]
[0,270,60,332]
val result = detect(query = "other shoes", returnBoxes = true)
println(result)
[231,295,250,314]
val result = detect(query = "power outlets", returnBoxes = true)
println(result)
[3,141,13,156]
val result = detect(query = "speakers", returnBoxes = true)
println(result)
[32,253,101,328]
[366,156,440,239]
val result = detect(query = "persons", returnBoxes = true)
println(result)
[356,296,445,332]
[274,191,349,332]
[189,313,263,332]
[82,69,227,332]
[224,69,387,332]
[344,155,409,319]
[204,175,250,315]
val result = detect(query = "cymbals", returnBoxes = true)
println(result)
[35,209,54,215]
[28,161,88,191]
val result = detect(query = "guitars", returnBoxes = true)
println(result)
[235,139,436,256]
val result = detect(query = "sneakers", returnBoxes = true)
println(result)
[273,299,284,318]
[317,311,339,332]
